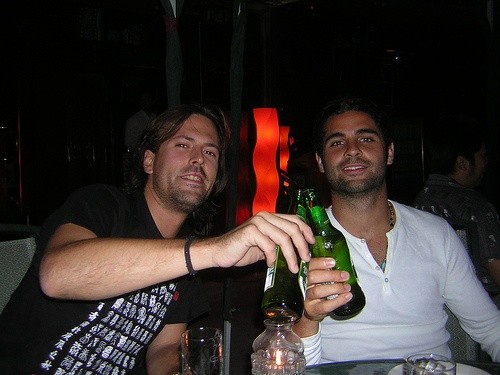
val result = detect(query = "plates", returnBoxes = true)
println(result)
[388,362,491,375]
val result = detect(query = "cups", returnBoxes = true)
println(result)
[406,353,457,375]
[179,327,224,375]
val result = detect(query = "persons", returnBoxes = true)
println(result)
[253,101,500,366]
[125,91,157,172]
[410,122,500,311]
[0,103,317,375]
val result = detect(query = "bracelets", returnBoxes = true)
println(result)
[185,236,198,276]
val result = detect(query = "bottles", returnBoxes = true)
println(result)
[250,320,307,375]
[260,188,310,323]
[303,188,366,320]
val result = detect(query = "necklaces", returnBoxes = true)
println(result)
[380,201,396,269]
